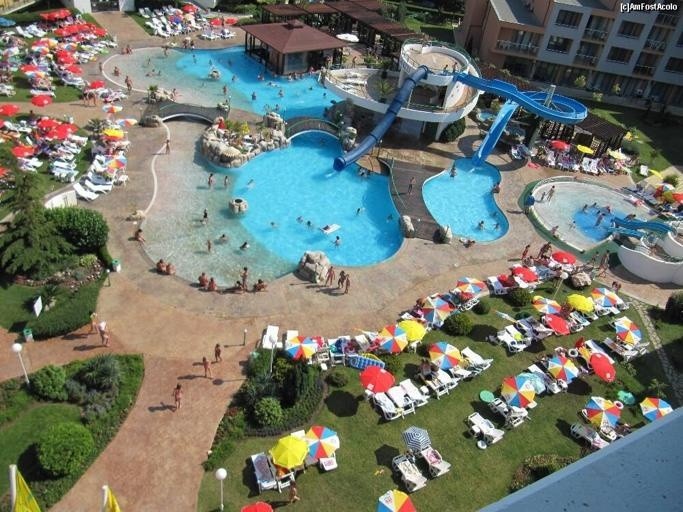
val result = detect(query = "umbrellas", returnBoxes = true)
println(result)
[374,489,416,511]
[639,397,673,421]
[645,168,683,205]
[585,395,622,429]
[267,435,305,471]
[549,140,569,160]
[609,151,628,171]
[360,362,396,395]
[285,335,320,361]
[241,500,273,512]
[302,425,341,457]
[400,425,431,452]
[0,8,137,178]
[575,144,594,166]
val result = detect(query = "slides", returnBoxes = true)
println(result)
[608,216,672,239]
[333,65,429,169]
[451,71,586,165]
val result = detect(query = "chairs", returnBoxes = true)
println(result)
[136,4,238,46]
[1,8,133,204]
[509,143,634,175]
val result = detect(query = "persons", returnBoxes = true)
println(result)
[520,245,530,259]
[134,228,143,241]
[449,166,455,179]
[478,220,483,229]
[337,271,345,287]
[99,320,111,349]
[287,479,300,504]
[325,266,334,287]
[582,202,636,228]
[223,176,228,190]
[231,281,243,292]
[212,343,222,363]
[549,223,560,238]
[540,192,547,201]
[535,241,553,259]
[144,40,330,115]
[525,191,535,215]
[165,139,172,154]
[199,207,211,224]
[333,235,341,246]
[344,342,356,367]
[218,234,227,240]
[85,310,98,336]
[546,185,555,202]
[351,56,357,67]
[198,272,208,290]
[253,278,266,291]
[493,223,501,231]
[492,184,496,192]
[569,220,576,232]
[207,277,217,291]
[490,182,501,195]
[165,263,176,274]
[545,141,551,148]
[206,238,212,253]
[201,357,212,379]
[240,267,248,292]
[156,258,168,275]
[407,176,415,197]
[239,240,248,249]
[172,383,182,408]
[554,151,618,176]
[155,1,237,39]
[207,172,215,188]
[344,274,351,292]
[366,337,378,356]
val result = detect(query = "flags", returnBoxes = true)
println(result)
[12,468,42,512]
[104,485,122,512]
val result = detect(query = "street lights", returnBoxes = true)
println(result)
[228,94,232,104]
[106,269,111,287]
[243,329,248,345]
[10,342,31,390]
[146,87,151,102]
[281,106,286,122]
[139,106,145,127]
[339,113,344,132]
[376,139,383,158]
[551,271,568,301]
[268,333,278,374]
[213,466,228,511]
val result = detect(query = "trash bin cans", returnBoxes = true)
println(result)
[112,258,120,272]
[22,329,33,341]
